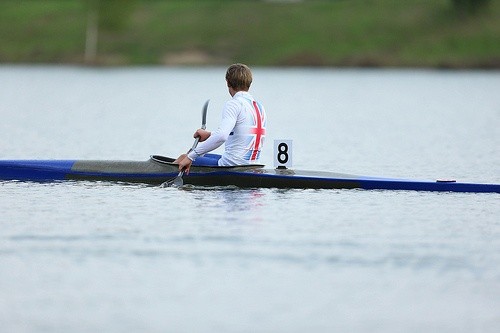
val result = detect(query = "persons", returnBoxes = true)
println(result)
[172,61,269,176]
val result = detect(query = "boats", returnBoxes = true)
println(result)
[0,154,499,193]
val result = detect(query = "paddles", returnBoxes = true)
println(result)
[160,98,211,187]
